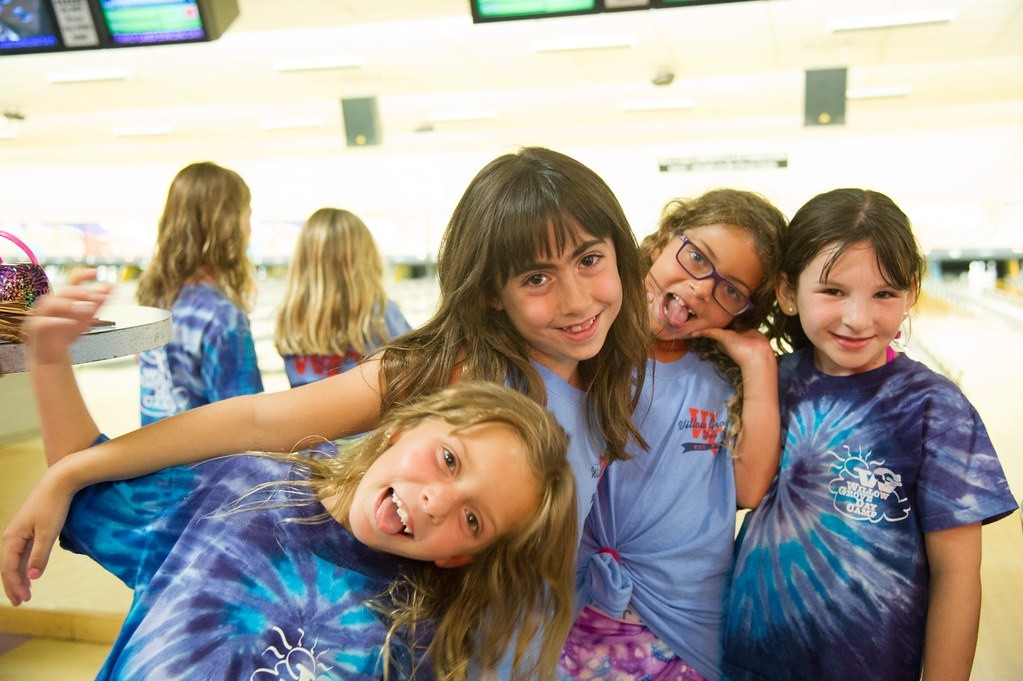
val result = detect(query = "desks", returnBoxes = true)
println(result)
[0,305,173,376]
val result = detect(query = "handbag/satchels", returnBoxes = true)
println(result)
[0,230,52,309]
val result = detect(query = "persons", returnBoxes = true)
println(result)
[720,188,1020,681]
[137,161,262,427]
[275,208,415,388]
[0,146,790,681]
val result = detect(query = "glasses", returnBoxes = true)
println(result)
[675,231,755,316]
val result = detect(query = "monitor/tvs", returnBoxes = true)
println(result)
[0,0,209,56]
